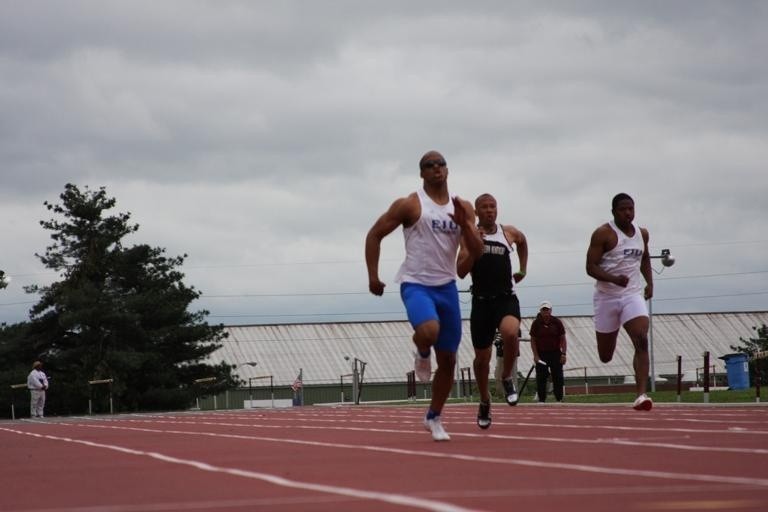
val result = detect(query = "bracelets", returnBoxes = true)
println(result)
[561,352,566,356]
[517,271,526,276]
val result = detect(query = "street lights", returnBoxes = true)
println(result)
[225,362,256,409]
[344,355,355,403]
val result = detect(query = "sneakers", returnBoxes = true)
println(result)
[500,372,518,407]
[413,347,432,386]
[476,391,493,430]
[422,415,451,442]
[631,393,655,411]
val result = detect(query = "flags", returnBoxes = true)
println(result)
[291,367,303,392]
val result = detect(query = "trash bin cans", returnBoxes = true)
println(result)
[718,353,749,390]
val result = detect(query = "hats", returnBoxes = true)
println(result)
[32,361,42,368]
[539,301,552,312]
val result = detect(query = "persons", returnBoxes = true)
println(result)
[493,323,522,398]
[585,191,654,410]
[365,147,486,441]
[456,191,529,429]
[27,361,49,419]
[528,300,568,403]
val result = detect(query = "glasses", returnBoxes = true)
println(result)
[423,158,446,168]
